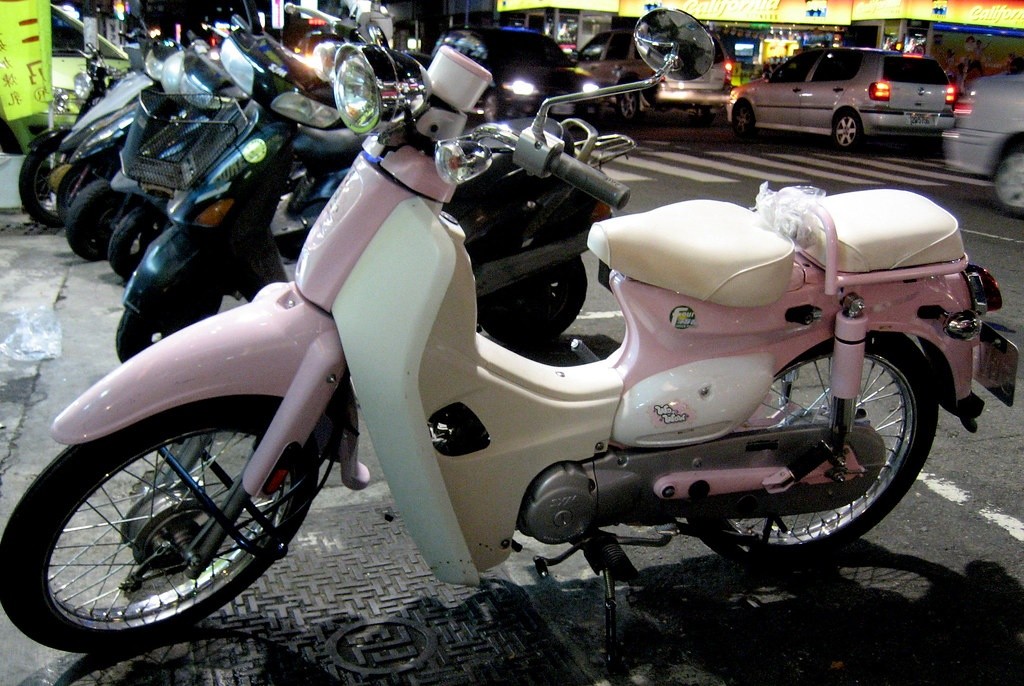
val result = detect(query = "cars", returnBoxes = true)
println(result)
[941,72,1024,227]
[430,27,734,125]
[0,6,133,155]
[727,47,957,149]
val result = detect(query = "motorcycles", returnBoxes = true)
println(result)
[17,14,642,363]
[0,3,1018,652]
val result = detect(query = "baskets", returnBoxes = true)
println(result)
[49,96,84,132]
[121,89,249,189]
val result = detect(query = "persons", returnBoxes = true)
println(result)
[546,19,572,42]
[761,55,784,78]
[933,34,1024,94]
